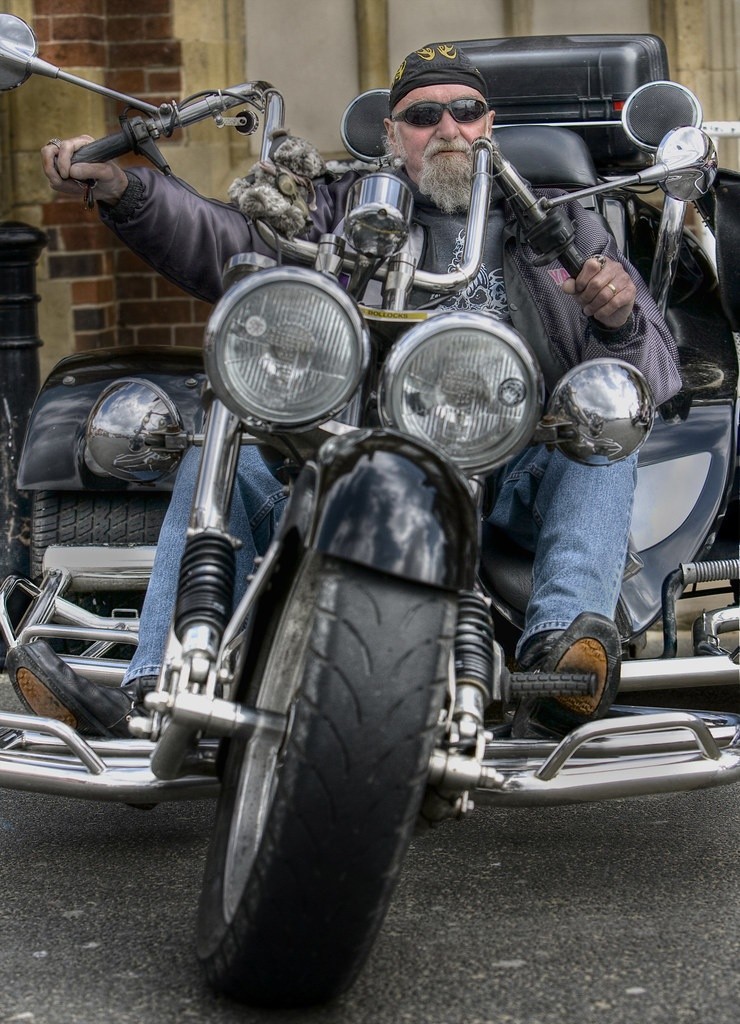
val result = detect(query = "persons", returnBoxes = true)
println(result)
[7,42,686,739]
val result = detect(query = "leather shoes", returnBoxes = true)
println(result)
[510,611,621,739]
[7,641,160,810]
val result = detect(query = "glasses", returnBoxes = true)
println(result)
[391,97,488,127]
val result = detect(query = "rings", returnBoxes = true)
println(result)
[607,283,617,296]
[591,254,606,269]
[45,136,62,149]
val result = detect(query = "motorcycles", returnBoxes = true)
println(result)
[0,13,740,1011]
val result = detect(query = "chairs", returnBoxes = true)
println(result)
[486,127,600,212]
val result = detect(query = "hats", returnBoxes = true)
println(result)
[388,42,489,113]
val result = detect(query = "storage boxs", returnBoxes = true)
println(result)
[423,31,669,172]
